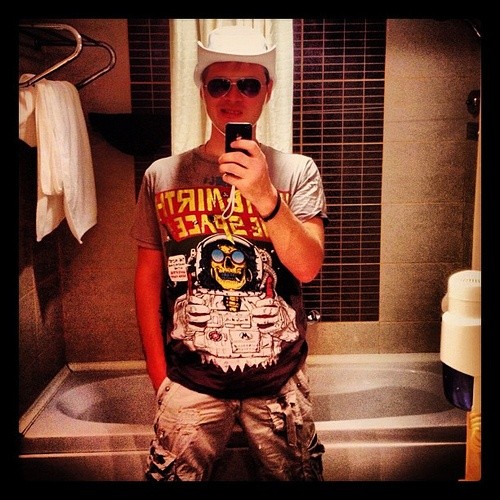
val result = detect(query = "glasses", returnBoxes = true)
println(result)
[204,76,266,99]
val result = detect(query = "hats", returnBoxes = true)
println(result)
[194,27,277,91]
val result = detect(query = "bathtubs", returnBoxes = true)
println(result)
[17,350,466,484]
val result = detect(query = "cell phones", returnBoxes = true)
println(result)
[225,122,253,157]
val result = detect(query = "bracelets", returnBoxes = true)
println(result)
[259,189,281,222]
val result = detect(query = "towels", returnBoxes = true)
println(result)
[17,72,98,245]
[168,17,294,158]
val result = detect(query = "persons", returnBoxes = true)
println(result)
[129,26,327,481]
